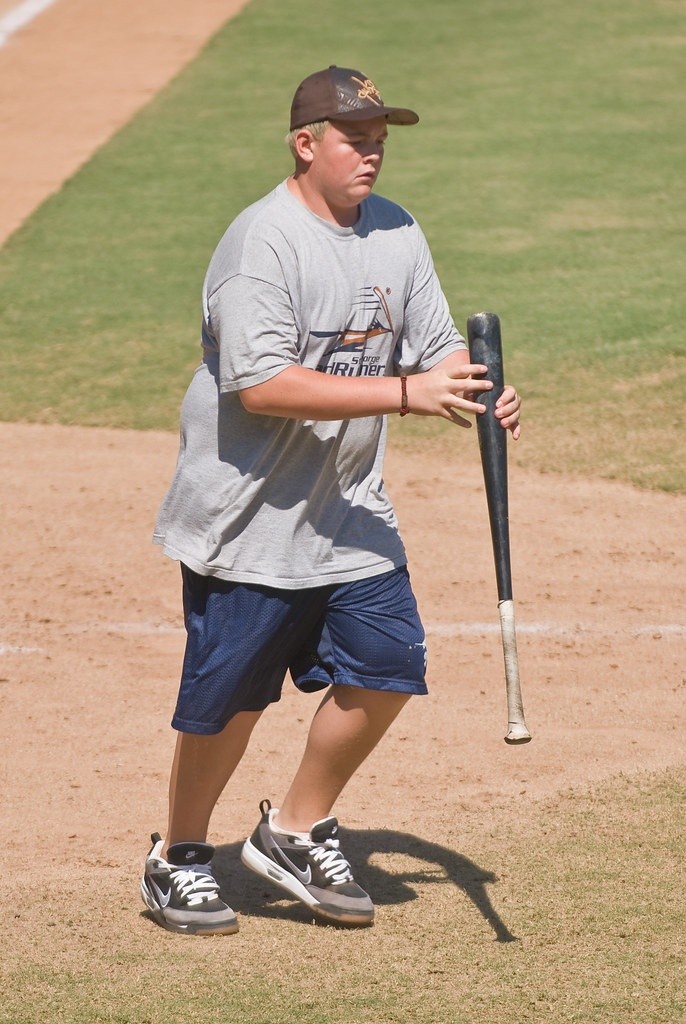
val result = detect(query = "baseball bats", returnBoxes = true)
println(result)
[467,310,535,746]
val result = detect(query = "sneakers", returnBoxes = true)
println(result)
[140,832,238,935]
[241,799,375,923]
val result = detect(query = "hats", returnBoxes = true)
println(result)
[289,65,419,126]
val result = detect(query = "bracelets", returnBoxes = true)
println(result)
[400,376,409,417]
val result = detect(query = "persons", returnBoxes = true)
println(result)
[137,65,522,936]
